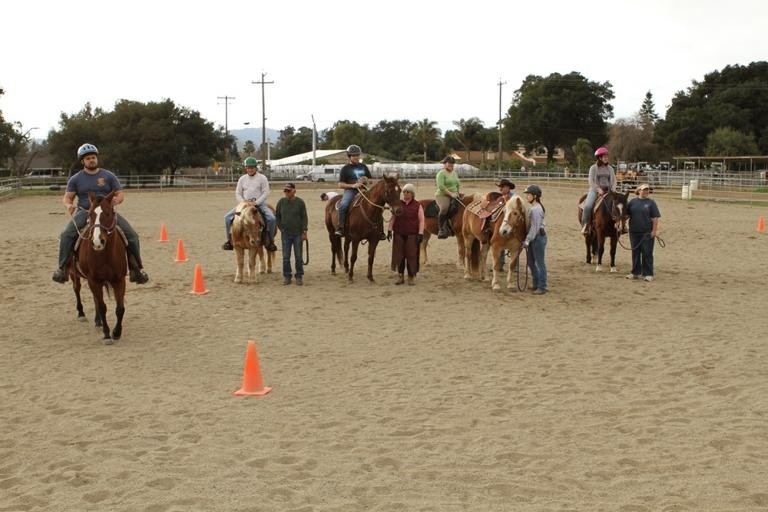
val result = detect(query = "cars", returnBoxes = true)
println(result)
[295,170,313,181]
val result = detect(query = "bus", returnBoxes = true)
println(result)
[22,167,66,178]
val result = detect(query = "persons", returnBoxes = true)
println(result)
[490,177,518,272]
[580,147,623,234]
[433,157,465,233]
[52,143,150,286]
[320,192,338,201]
[219,158,278,252]
[625,183,662,281]
[335,144,372,237]
[522,183,547,295]
[386,183,425,286]
[275,184,309,285]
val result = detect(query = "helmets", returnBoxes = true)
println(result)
[444,156,455,164]
[347,145,361,157]
[245,157,258,167]
[78,143,98,161]
[594,148,609,157]
[523,184,541,197]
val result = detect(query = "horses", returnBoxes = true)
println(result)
[68,189,128,346]
[418,193,474,270]
[615,165,648,181]
[230,198,277,285]
[462,194,527,293]
[325,171,404,287]
[577,185,630,273]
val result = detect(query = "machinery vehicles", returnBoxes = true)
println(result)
[612,160,653,195]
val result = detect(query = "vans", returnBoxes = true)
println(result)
[311,166,342,183]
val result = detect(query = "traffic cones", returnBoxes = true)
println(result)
[188,263,210,295]
[234,340,272,395]
[174,239,189,262]
[158,223,171,242]
[756,217,766,233]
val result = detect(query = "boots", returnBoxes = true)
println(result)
[395,273,404,284]
[438,214,448,237]
[408,274,416,285]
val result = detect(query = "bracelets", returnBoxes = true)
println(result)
[302,231,306,234]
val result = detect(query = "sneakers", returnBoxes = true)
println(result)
[644,275,654,282]
[335,229,344,237]
[532,289,545,294]
[52,273,69,283]
[224,240,233,250]
[281,280,291,285]
[296,278,303,286]
[582,223,591,234]
[130,271,149,282]
[626,274,642,279]
[269,244,277,252]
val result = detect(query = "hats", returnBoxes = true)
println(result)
[495,179,515,189]
[639,183,650,191]
[284,183,295,191]
[402,184,416,197]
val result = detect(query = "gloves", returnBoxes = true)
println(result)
[387,231,392,241]
[415,234,423,244]
